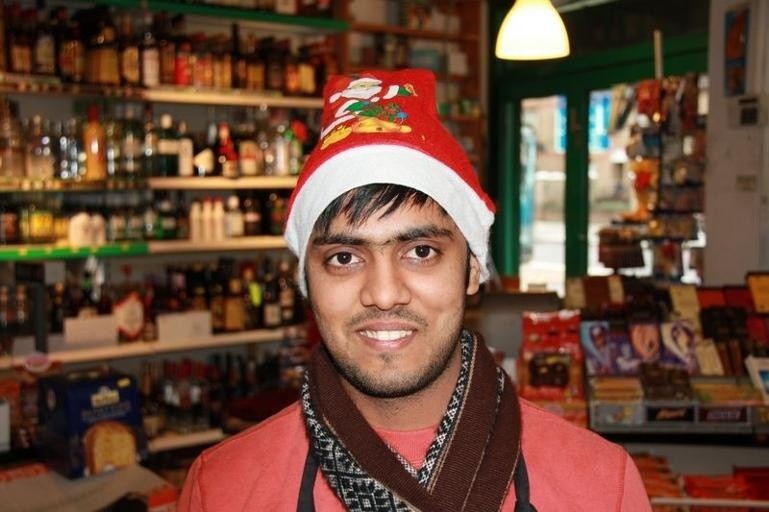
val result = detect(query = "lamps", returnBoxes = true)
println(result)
[491,1,571,62]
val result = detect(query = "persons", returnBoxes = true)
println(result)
[176,184,650,511]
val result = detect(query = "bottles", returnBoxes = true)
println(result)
[131,351,260,438]
[0,1,328,353]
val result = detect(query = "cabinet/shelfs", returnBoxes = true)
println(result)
[524,283,768,511]
[4,1,477,470]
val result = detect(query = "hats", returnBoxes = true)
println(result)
[283,66,497,298]
